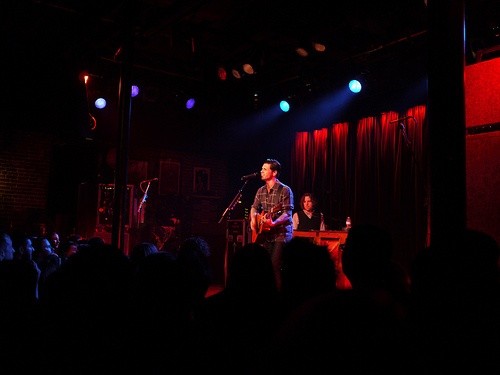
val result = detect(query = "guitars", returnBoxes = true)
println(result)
[250,207,282,244]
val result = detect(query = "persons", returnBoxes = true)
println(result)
[0,198,500,375]
[250,158,293,286]
[292,193,326,230]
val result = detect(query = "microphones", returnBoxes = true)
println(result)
[242,172,258,180]
[142,178,158,185]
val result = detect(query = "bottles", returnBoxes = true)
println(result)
[320,216,325,231]
[346,216,351,231]
